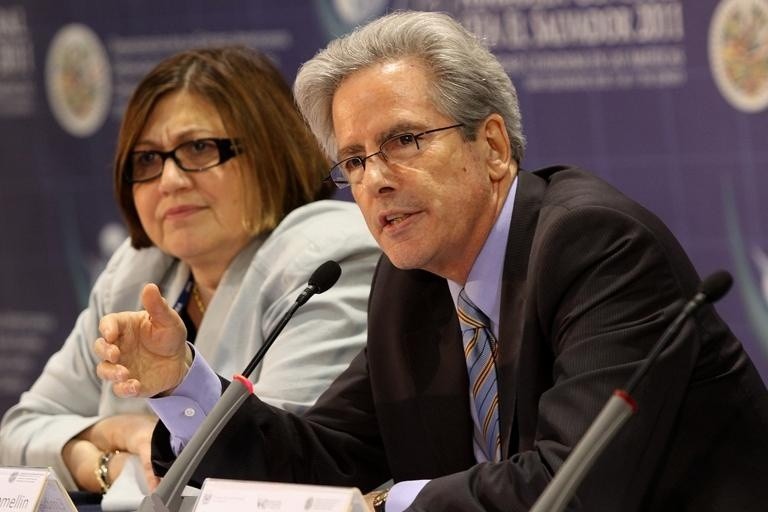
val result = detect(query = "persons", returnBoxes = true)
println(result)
[0,42,384,503]
[90,8,768,512]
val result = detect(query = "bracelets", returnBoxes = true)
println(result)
[94,449,117,494]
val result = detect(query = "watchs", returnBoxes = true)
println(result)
[371,490,390,512]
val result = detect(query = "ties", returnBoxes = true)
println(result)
[456,289,502,466]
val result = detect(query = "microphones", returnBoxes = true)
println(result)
[139,259,342,512]
[528,269,734,512]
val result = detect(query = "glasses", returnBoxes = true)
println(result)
[129,136,247,183]
[328,123,464,190]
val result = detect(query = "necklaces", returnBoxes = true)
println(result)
[191,283,207,315]
